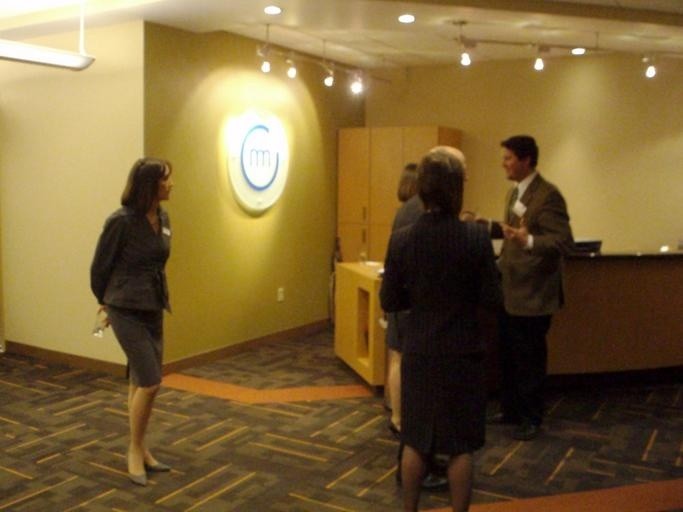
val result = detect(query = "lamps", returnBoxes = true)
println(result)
[452,20,656,79]
[255,24,362,98]
[0,3,96,72]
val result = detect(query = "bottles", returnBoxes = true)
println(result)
[90,308,108,338]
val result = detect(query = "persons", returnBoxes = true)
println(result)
[88,156,177,489]
[464,133,577,442]
[379,144,508,512]
[377,159,430,439]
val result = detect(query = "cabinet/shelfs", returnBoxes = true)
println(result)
[337,125,461,263]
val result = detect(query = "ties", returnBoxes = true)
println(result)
[508,188,518,223]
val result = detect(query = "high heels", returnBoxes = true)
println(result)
[143,460,171,473]
[125,449,148,488]
[389,421,402,438]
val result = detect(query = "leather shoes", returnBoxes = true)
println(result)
[511,406,543,440]
[397,468,449,492]
[425,456,448,477]
